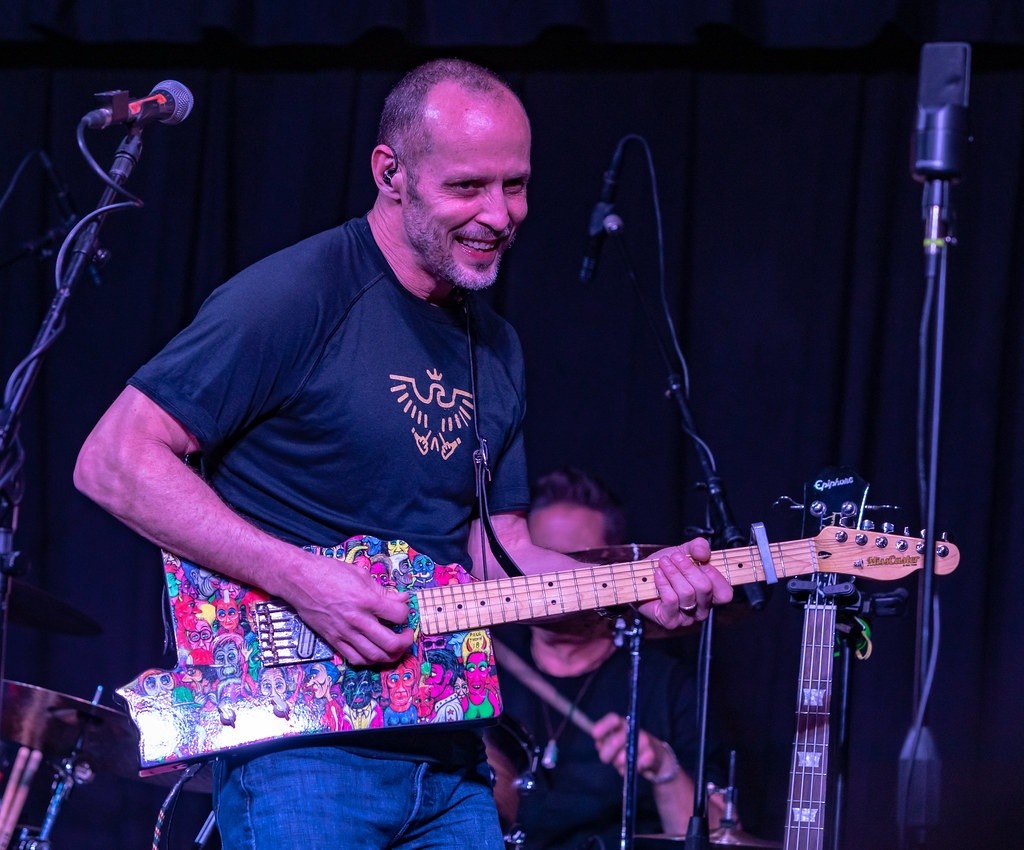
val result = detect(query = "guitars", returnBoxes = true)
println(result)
[112,494,963,783]
[768,463,902,850]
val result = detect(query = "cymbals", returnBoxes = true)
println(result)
[632,827,784,850]
[1,678,144,774]
[531,542,757,640]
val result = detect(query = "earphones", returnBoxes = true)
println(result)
[383,168,397,184]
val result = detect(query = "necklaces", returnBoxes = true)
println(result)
[541,635,615,770]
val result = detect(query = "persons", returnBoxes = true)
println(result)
[73,60,733,850]
[481,469,741,850]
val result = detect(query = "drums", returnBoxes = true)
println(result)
[481,710,545,850]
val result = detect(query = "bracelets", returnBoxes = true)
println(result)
[640,742,679,785]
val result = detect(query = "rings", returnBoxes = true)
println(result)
[680,603,700,614]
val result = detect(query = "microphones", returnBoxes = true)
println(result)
[82,80,193,131]
[911,41,974,279]
[580,144,625,281]
